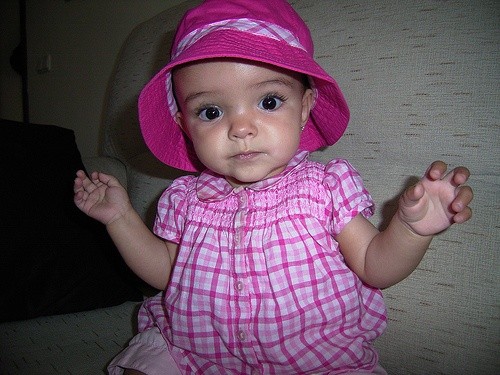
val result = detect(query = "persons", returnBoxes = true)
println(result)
[73,0,474,374]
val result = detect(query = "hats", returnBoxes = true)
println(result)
[138,0,350,173]
